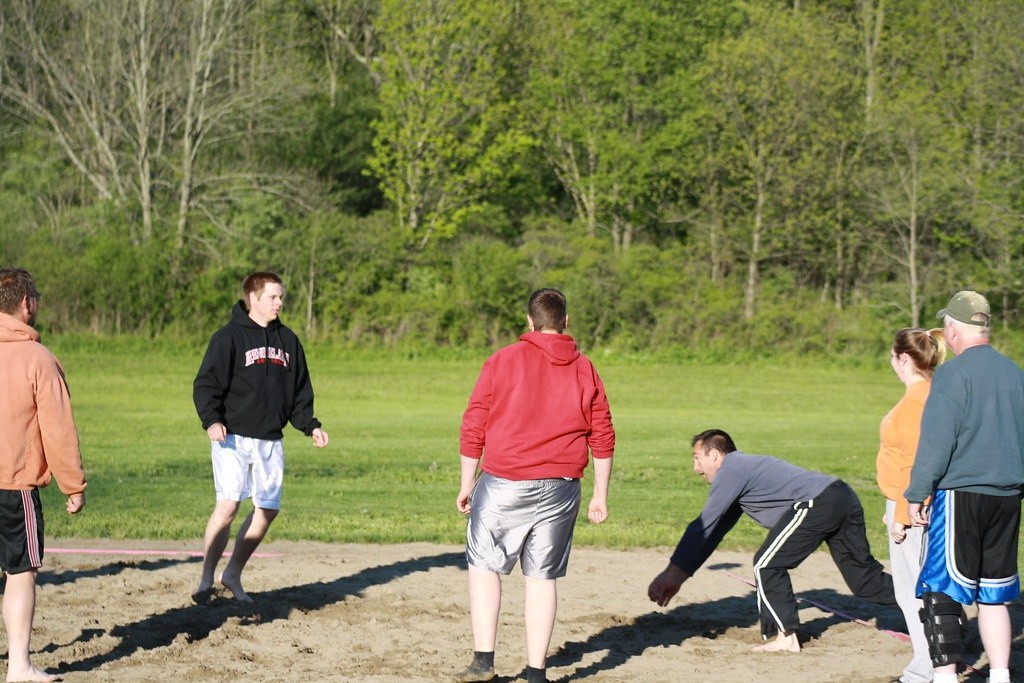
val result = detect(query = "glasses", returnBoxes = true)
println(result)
[22,291,43,303]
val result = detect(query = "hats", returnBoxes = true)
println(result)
[936,290,992,327]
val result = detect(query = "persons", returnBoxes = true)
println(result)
[193,271,330,603]
[0,270,85,683]
[905,291,1024,683]
[878,325,934,683]
[647,430,899,653]
[454,287,617,683]
